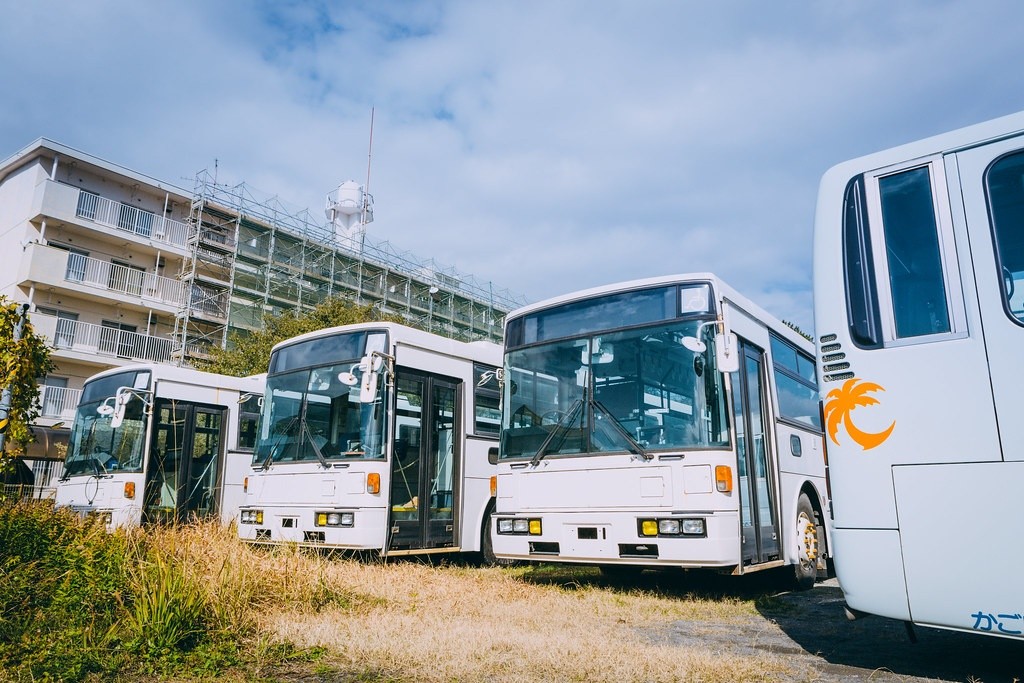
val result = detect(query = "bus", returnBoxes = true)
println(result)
[476,273,833,590]
[812,111,1024,644]
[237,321,714,568]
[53,363,531,536]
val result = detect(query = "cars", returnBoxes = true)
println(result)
[0,459,35,498]
